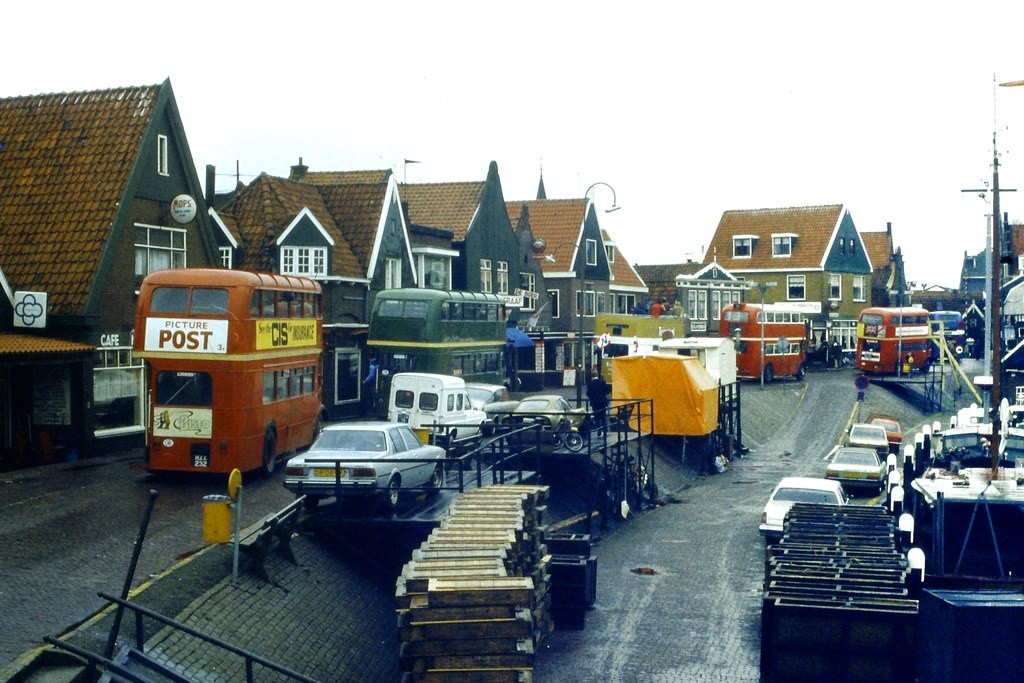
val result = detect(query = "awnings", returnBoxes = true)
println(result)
[505,327,536,347]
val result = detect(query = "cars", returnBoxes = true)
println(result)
[759,476,854,546]
[864,415,903,454]
[281,420,448,513]
[843,423,890,460]
[461,382,508,414]
[824,447,889,495]
[484,394,587,438]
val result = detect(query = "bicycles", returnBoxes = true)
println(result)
[532,416,584,452]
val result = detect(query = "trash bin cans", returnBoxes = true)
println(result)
[202,494,231,545]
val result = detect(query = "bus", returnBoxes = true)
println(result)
[127,268,335,477]
[366,288,508,418]
[718,303,808,384]
[591,312,690,390]
[855,307,933,375]
[929,311,965,364]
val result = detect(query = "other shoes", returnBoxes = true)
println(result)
[597,434,610,440]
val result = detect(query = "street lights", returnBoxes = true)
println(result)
[545,242,585,409]
[582,182,622,239]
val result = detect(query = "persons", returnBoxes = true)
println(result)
[586,372,610,436]
[831,340,842,368]
[362,353,380,407]
[651,300,666,317]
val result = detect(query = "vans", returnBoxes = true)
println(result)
[386,372,487,448]
